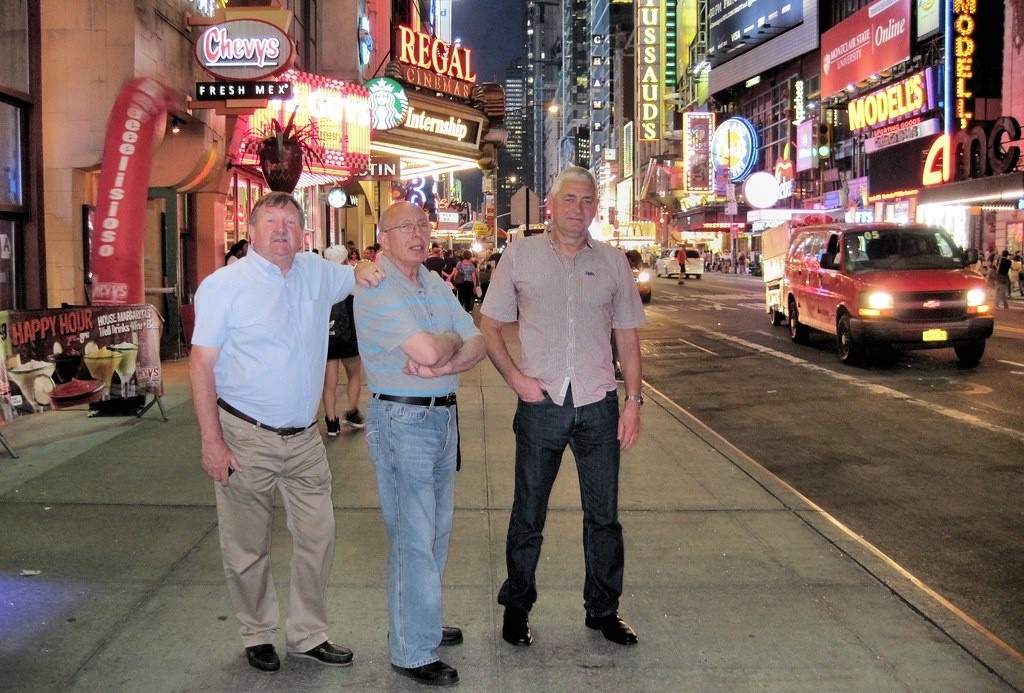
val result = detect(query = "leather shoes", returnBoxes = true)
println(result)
[387,626,463,645]
[246,644,280,673]
[391,660,460,685]
[287,640,354,666]
[585,613,637,646]
[502,608,533,647]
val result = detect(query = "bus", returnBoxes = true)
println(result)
[507,223,546,243]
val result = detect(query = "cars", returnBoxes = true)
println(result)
[631,262,651,302]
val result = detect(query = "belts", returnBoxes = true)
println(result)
[372,393,457,407]
[216,397,319,436]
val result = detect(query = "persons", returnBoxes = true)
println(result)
[353,201,485,685]
[188,192,385,674]
[939,245,1024,311]
[909,240,931,256]
[226,234,763,436]
[478,165,648,647]
[833,236,870,265]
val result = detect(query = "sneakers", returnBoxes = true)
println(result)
[344,409,365,429]
[324,414,341,437]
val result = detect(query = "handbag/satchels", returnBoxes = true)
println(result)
[454,260,464,287]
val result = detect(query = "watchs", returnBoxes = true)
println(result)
[623,394,644,406]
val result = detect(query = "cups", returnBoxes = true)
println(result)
[83,351,123,401]
[8,362,56,413]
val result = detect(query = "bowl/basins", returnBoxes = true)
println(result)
[49,379,105,411]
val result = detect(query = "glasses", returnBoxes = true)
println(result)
[384,222,430,235]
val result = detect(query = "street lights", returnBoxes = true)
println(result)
[520,103,560,237]
[602,198,619,246]
[697,148,736,275]
[493,176,516,248]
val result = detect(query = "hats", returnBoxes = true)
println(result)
[324,245,348,265]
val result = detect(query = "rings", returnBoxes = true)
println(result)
[373,269,379,275]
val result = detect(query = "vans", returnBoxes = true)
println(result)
[781,222,996,365]
[653,248,704,279]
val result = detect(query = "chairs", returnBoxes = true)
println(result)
[821,234,841,271]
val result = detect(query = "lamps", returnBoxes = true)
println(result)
[808,102,828,109]
[724,45,749,50]
[494,176,517,183]
[870,74,890,81]
[846,84,864,91]
[170,119,181,135]
[741,35,768,40]
[708,55,732,59]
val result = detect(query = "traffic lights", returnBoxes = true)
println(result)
[546,209,551,219]
[812,123,830,158]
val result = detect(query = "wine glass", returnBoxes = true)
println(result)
[110,344,139,397]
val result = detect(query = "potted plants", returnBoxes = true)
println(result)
[237,104,329,193]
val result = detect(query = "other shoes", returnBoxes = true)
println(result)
[1004,308,1009,311]
[469,311,472,313]
[994,304,1001,311]
